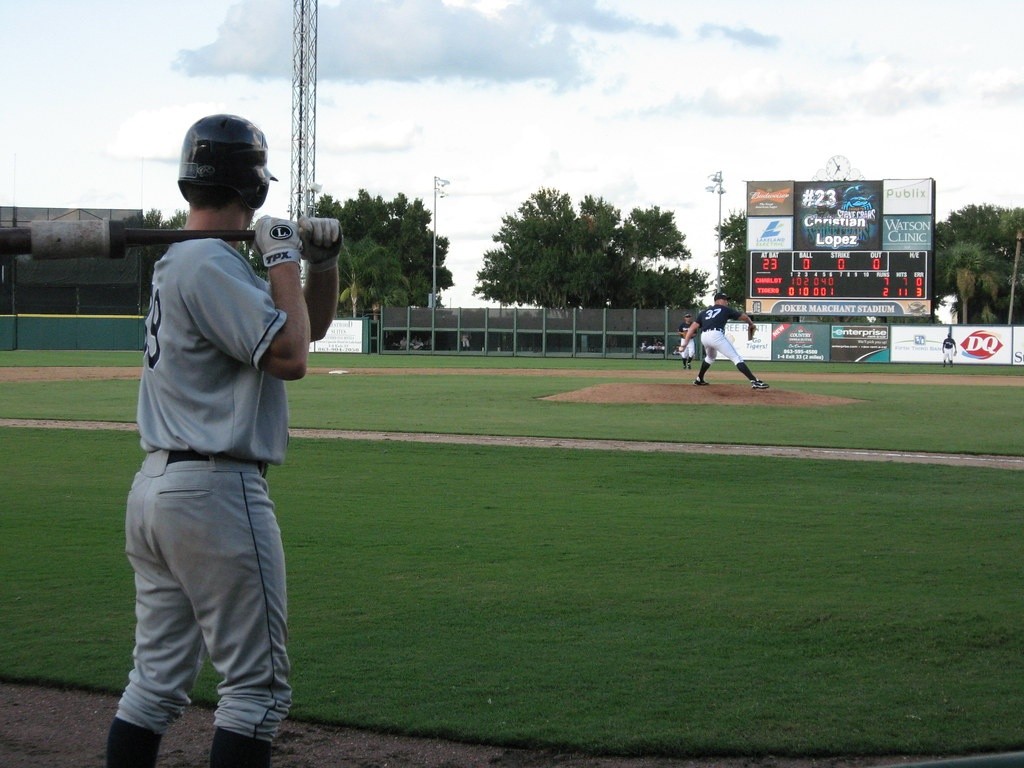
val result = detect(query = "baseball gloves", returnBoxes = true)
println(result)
[748,325,756,341]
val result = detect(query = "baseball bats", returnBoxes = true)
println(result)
[0,219,306,261]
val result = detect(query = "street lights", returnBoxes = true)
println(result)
[706,170,726,294]
[432,176,451,353]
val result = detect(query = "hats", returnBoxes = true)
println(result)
[714,293,731,300]
[683,313,692,318]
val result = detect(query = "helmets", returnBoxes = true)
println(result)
[178,114,278,210]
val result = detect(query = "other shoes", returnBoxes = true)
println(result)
[687,362,691,369]
[683,364,686,369]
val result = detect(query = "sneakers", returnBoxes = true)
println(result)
[750,378,769,388]
[693,377,709,385]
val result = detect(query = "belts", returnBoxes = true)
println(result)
[166,450,210,463]
[704,328,720,332]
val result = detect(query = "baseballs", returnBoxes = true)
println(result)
[679,347,684,352]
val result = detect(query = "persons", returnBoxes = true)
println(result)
[462,333,471,351]
[105,114,343,768]
[942,333,957,368]
[679,293,770,389]
[399,335,432,350]
[639,337,665,353]
[677,314,697,369]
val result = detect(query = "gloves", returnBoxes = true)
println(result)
[298,215,342,273]
[250,215,303,270]
